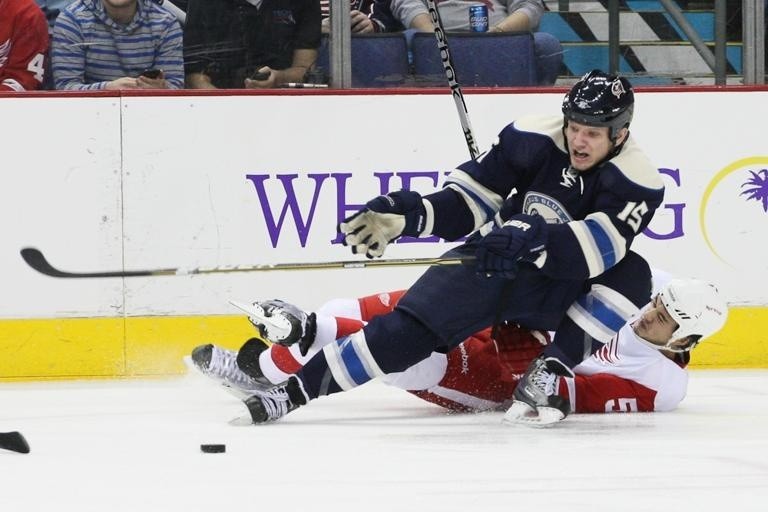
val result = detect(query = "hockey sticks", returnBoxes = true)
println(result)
[20,248,481,278]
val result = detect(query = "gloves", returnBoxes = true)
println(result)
[334,191,426,259]
[471,213,551,281]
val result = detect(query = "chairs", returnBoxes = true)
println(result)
[304,29,542,92]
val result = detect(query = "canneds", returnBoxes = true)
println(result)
[469,4,488,31]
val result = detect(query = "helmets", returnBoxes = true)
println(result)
[562,69,635,141]
[659,277,727,344]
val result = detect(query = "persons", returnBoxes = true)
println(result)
[0,1,74,92]
[183,2,322,88]
[243,69,667,426]
[320,1,401,34]
[389,1,562,88]
[190,273,728,417]
[51,0,184,91]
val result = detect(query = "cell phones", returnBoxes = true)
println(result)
[250,64,269,81]
[133,67,162,81]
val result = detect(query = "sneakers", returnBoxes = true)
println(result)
[247,300,310,347]
[512,354,576,419]
[191,338,312,423]
